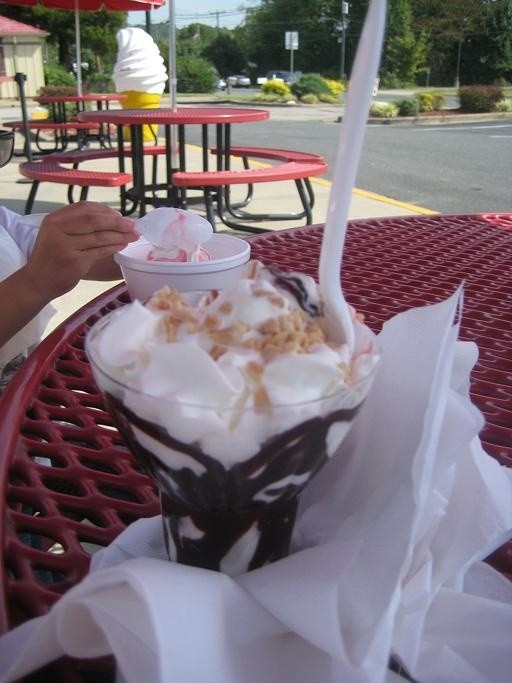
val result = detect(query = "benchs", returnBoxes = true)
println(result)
[3,115,114,156]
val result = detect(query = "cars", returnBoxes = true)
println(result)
[215,68,300,91]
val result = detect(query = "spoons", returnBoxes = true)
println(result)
[15,206,213,253]
[314,0,389,350]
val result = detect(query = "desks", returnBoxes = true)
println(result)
[78,106,269,233]
[33,93,129,151]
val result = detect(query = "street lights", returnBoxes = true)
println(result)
[316,16,348,79]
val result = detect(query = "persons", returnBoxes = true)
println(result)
[0,199,162,599]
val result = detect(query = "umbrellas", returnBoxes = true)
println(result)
[1,0,168,115]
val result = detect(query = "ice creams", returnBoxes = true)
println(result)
[112,26,169,141]
[134,208,212,263]
[90,260,377,577]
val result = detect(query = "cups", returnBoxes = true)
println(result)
[84,293,382,571]
[111,233,250,301]
